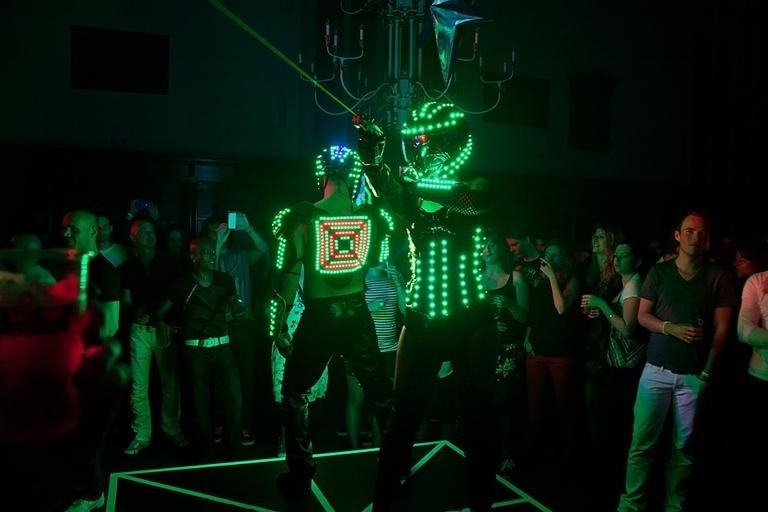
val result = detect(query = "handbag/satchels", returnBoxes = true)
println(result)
[605,327,646,369]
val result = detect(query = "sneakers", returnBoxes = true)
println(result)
[65,490,105,512]
[166,431,189,447]
[240,428,255,447]
[124,439,146,455]
[213,426,224,443]
[277,472,310,495]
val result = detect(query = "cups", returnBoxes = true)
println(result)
[686,315,704,343]
[586,306,600,319]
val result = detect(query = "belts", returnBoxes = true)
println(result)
[185,335,230,349]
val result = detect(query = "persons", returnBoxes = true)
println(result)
[51,206,124,342]
[374,223,499,511]
[97,213,131,271]
[203,209,268,448]
[269,141,397,512]
[156,227,190,267]
[104,216,192,457]
[505,210,766,510]
[478,235,531,477]
[163,236,248,465]
[344,261,406,466]
[270,266,332,459]
[1,233,58,288]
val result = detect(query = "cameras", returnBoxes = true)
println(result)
[226,209,237,231]
